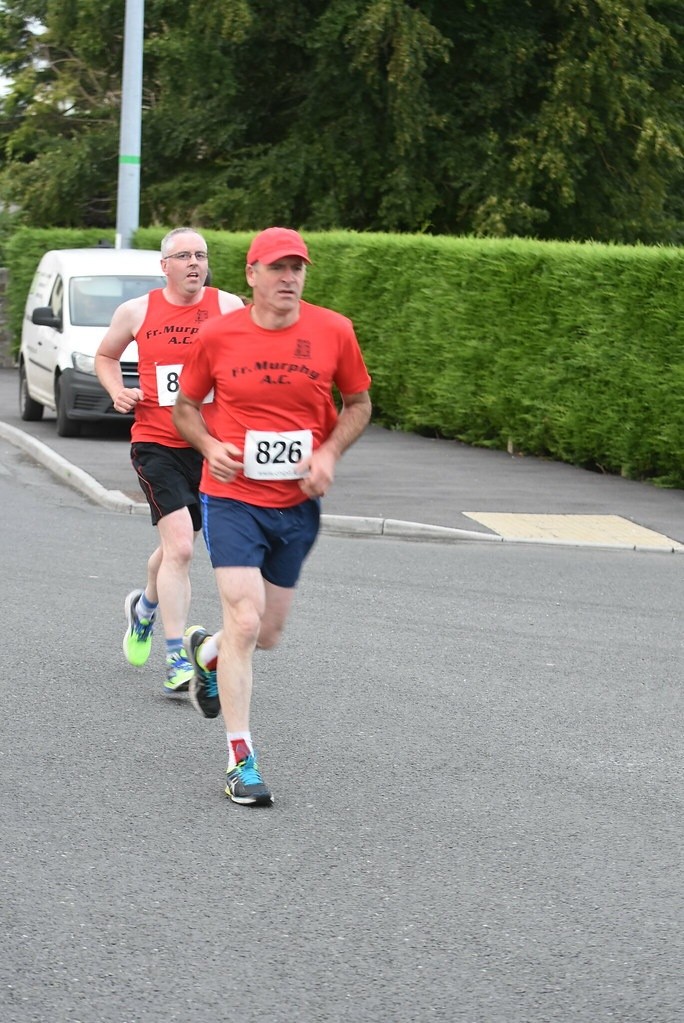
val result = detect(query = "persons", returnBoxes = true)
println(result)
[171,229,372,807]
[93,228,248,692]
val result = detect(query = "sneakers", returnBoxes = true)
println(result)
[183,625,221,718]
[225,759,273,805]
[123,587,158,666]
[163,650,196,692]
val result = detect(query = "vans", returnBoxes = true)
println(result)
[19,247,211,436]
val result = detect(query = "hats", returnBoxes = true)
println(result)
[247,228,313,268]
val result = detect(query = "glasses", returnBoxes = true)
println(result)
[164,251,208,261]
